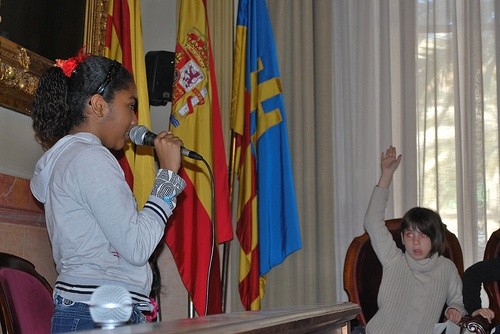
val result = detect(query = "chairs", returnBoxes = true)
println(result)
[344,217,463,334]
[0,252,54,334]
[462,228,500,334]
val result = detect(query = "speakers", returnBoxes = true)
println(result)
[145,50,175,106]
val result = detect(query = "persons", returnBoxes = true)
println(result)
[462,258,500,334]
[29,45,186,334]
[364,145,465,334]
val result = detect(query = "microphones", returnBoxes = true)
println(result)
[130,125,203,161]
[87,283,133,327]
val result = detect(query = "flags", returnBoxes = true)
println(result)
[229,0,303,311]
[102,0,158,211]
[164,0,234,317]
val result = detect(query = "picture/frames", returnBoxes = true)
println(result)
[0,0,110,116]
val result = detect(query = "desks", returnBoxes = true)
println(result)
[63,302,361,334]
